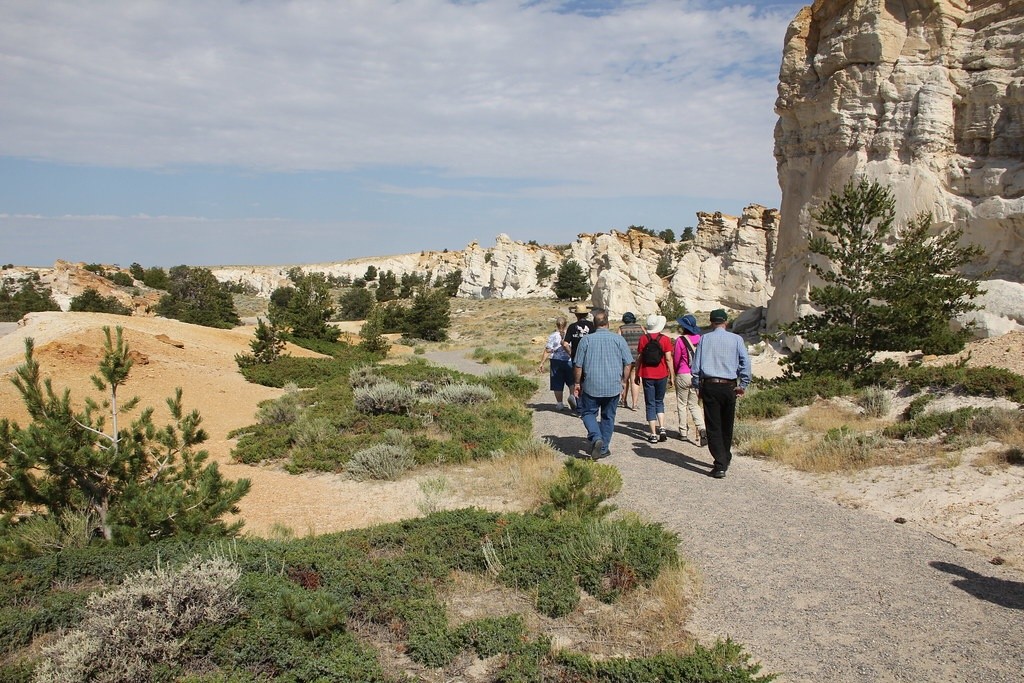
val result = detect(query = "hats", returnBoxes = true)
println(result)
[676,315,700,335]
[710,309,728,322]
[572,304,590,313]
[646,315,666,333]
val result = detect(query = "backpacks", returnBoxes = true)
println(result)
[641,333,665,367]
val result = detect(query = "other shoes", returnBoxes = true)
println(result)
[592,440,603,461]
[600,449,611,458]
[555,403,564,411]
[677,433,687,440]
[648,434,657,442]
[568,396,577,411]
[632,404,637,410]
[660,428,666,441]
[617,398,627,408]
[699,429,708,446]
[711,469,725,477]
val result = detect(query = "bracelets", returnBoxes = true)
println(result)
[575,382,580,384]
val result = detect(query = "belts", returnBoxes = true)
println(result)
[705,378,736,383]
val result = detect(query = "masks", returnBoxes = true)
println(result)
[679,328,685,333]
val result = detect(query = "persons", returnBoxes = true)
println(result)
[562,302,599,418]
[667,314,709,446]
[573,311,634,460]
[615,311,646,411]
[633,313,675,444]
[538,316,575,411]
[690,308,751,478]
